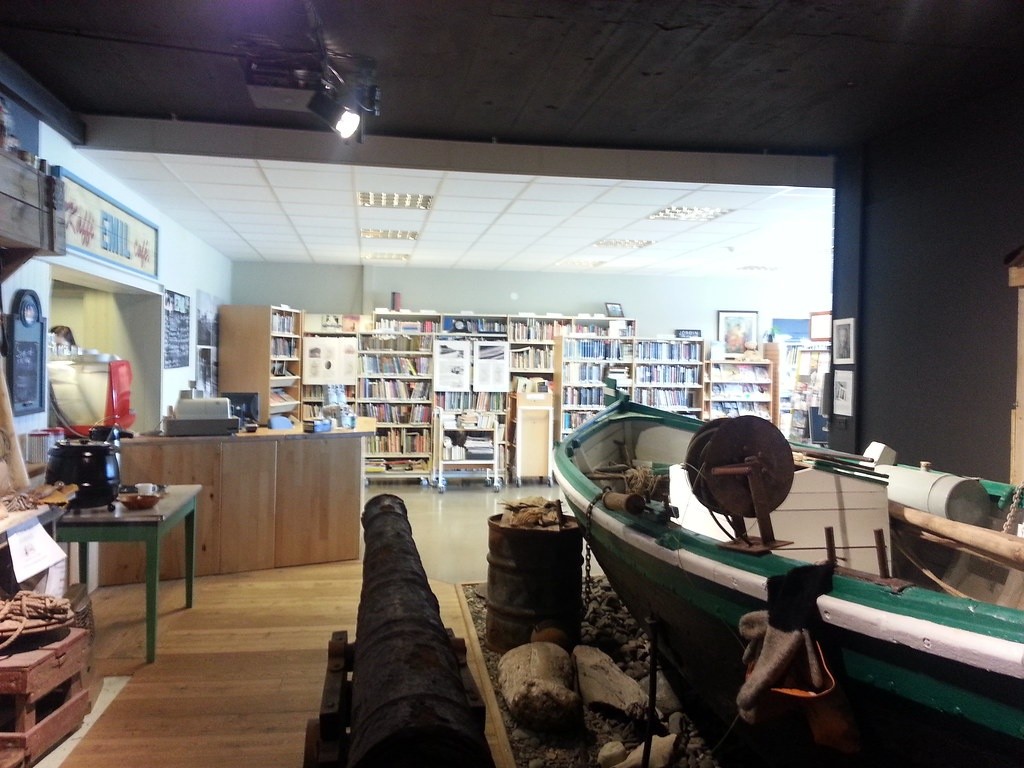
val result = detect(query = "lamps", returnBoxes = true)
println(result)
[303,85,362,141]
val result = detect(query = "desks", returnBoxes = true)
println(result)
[44,484,204,664]
[0,626,92,768]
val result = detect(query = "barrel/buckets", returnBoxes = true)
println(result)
[484,513,583,654]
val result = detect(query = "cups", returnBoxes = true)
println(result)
[135,482,158,495]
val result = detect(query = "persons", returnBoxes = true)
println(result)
[47,325,76,428]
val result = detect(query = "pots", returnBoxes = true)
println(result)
[44,438,121,513]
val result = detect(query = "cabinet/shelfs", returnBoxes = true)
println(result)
[554,337,635,442]
[215,305,300,427]
[509,316,636,380]
[436,405,502,496]
[504,392,554,486]
[301,314,373,423]
[357,332,433,481]
[636,337,705,422]
[374,311,507,343]
[709,362,780,430]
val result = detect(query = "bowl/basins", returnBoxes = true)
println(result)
[116,494,160,510]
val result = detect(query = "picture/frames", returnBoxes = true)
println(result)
[606,301,624,318]
[675,329,701,338]
[717,309,759,359]
[809,310,832,340]
[832,370,855,419]
[834,318,856,366]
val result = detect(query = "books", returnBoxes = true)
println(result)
[271,313,296,401]
[563,339,632,429]
[365,459,427,472]
[357,354,433,375]
[303,385,355,418]
[510,320,633,341]
[366,428,431,453]
[375,319,441,349]
[362,334,420,351]
[447,320,507,355]
[633,341,698,417]
[435,392,506,472]
[359,402,430,423]
[357,378,430,400]
[512,344,554,392]
[712,365,772,418]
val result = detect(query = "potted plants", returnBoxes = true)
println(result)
[763,328,780,343]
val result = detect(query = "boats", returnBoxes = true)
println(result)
[549,375,1023,768]
